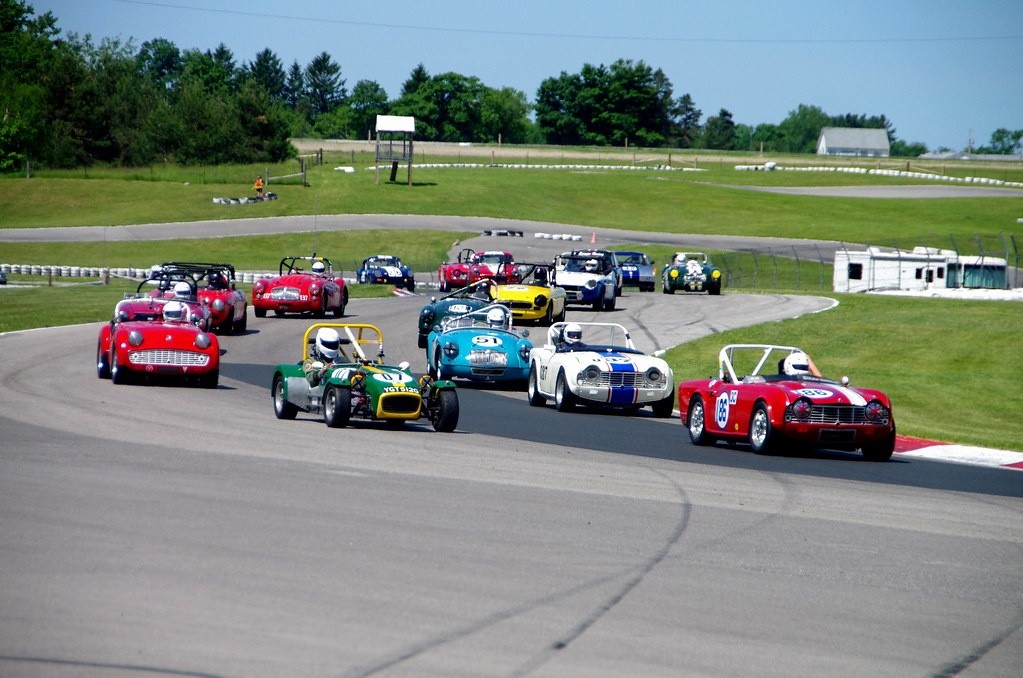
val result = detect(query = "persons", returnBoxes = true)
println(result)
[305,328,347,387]
[677,254,686,264]
[163,303,182,320]
[312,261,325,277]
[487,308,506,330]
[388,261,396,266]
[630,254,640,264]
[476,283,491,299]
[784,353,810,376]
[528,267,550,286]
[563,324,584,347]
[208,275,227,290]
[253,175,265,197]
[174,282,191,300]
[581,259,600,274]
[471,254,479,263]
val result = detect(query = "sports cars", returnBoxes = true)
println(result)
[661,251,722,296]
[95,278,221,389]
[425,303,537,388]
[525,322,675,418]
[417,247,657,349]
[136,260,249,337]
[270,323,460,433]
[252,256,348,318]
[677,343,896,461]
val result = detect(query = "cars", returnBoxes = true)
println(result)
[355,254,416,293]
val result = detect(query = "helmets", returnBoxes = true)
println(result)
[387,260,396,267]
[630,255,641,263]
[470,254,479,263]
[585,259,598,271]
[783,352,810,376]
[316,328,340,358]
[162,302,182,321]
[677,254,687,266]
[487,308,505,330]
[534,268,547,280]
[207,271,222,287]
[311,262,325,276]
[174,282,191,300]
[510,271,522,284]
[564,323,582,344]
[479,282,490,294]
